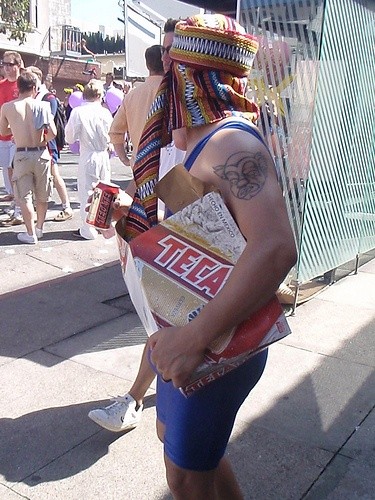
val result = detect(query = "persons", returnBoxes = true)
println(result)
[48,38,131,159]
[84,13,298,500]
[19,66,72,221]
[0,51,21,203]
[0,72,56,244]
[88,18,189,432]
[63,84,113,240]
[108,45,162,172]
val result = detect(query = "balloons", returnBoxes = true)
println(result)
[104,88,123,113]
[68,91,83,108]
[68,139,79,154]
[108,150,116,158]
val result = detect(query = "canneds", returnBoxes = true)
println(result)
[85,180,120,230]
